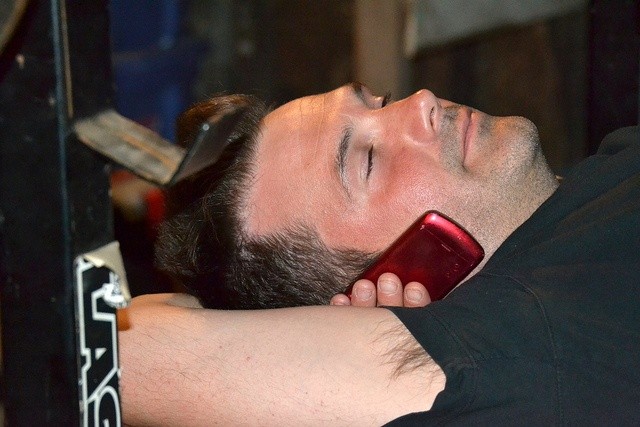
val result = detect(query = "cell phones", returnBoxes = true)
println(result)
[343,209,485,301]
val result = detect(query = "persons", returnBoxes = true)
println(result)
[116,81,640,426]
